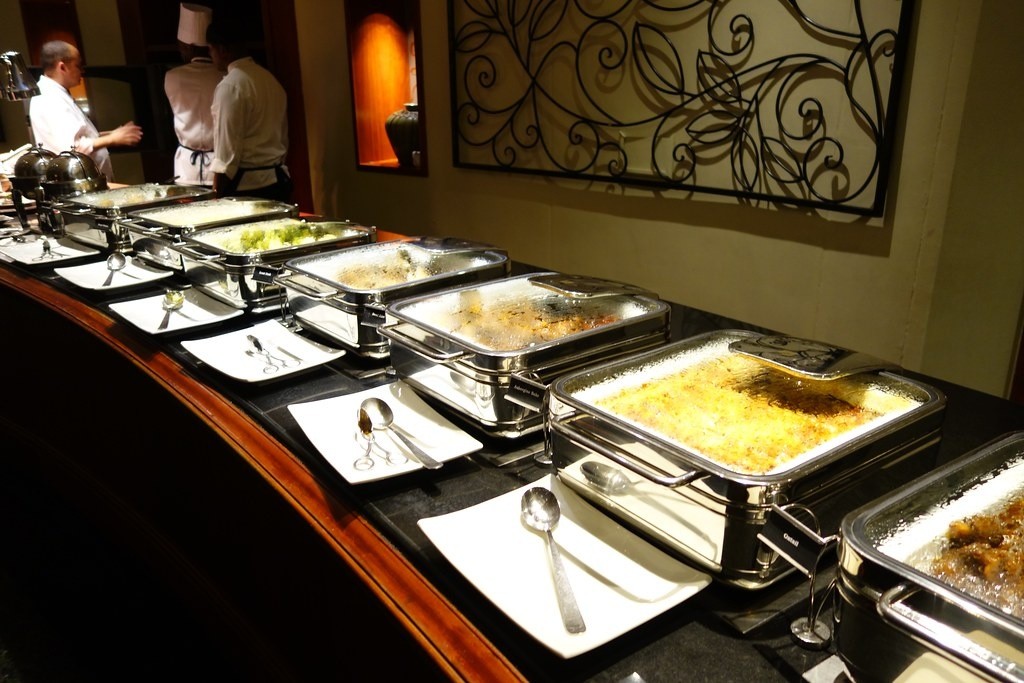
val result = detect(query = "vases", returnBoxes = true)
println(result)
[384,103,425,168]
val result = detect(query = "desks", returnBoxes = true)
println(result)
[0,213,1024,683]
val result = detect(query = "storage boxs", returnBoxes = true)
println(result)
[41,180,218,252]
[118,196,300,270]
[179,219,378,308]
[282,235,512,362]
[833,430,1024,683]
[384,270,673,438]
[546,328,949,593]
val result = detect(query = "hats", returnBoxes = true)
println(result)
[177,3,212,47]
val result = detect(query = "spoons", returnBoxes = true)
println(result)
[358,396,446,473]
[579,463,701,497]
[155,289,185,330]
[450,372,479,397]
[101,251,127,286]
[519,485,587,633]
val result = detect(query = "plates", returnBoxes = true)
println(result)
[53,255,173,291]
[0,227,30,238]
[179,318,346,384]
[415,469,716,661]
[0,194,37,206]
[0,237,101,266]
[108,287,245,335]
[561,440,733,573]
[885,648,996,683]
[408,362,498,423]
[287,378,484,485]
[294,302,398,348]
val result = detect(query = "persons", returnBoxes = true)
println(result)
[164,8,295,205]
[29,41,143,184]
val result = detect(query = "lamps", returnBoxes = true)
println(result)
[0,51,42,102]
